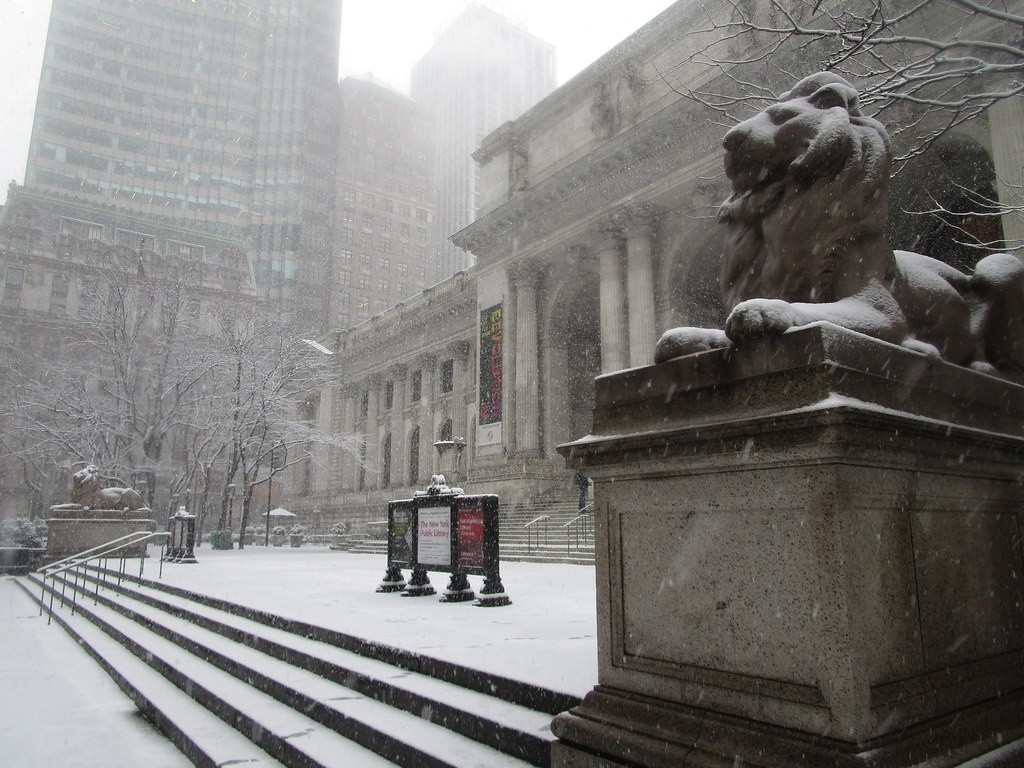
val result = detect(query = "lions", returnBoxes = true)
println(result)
[651,70,1023,383]
[50,466,153,517]
